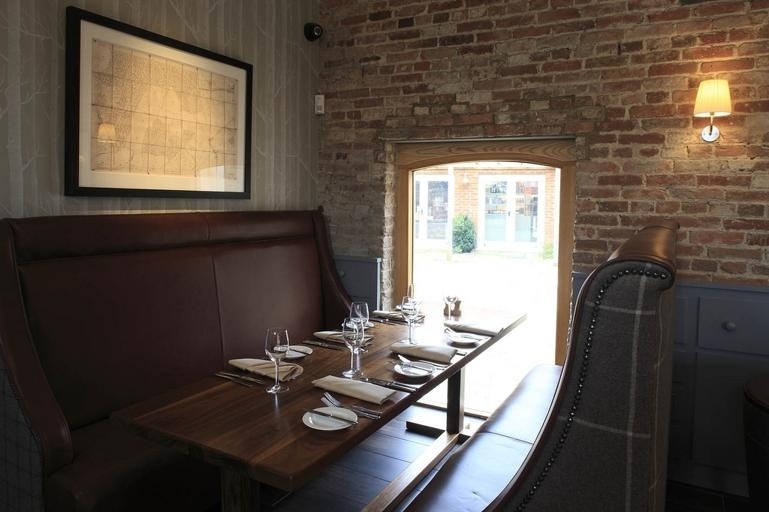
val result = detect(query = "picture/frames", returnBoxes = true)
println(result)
[64,5,253,200]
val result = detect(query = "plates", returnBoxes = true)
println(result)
[272,344,314,360]
[446,330,482,346]
[302,406,358,431]
[394,361,436,378]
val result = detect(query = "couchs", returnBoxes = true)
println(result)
[400,218,682,512]
[0,209,359,512]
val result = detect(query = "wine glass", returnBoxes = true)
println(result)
[443,291,457,324]
[342,301,369,378]
[264,326,290,395]
[401,295,420,345]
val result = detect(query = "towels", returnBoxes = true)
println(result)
[227,310,504,407]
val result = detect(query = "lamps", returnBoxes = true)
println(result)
[693,79,733,142]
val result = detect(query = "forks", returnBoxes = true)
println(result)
[321,391,383,422]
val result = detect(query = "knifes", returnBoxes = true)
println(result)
[370,376,420,393]
[301,339,342,352]
[214,368,263,388]
[305,406,356,425]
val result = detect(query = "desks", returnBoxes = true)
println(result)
[743,373,769,512]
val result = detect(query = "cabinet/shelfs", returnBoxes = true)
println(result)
[571,271,769,499]
